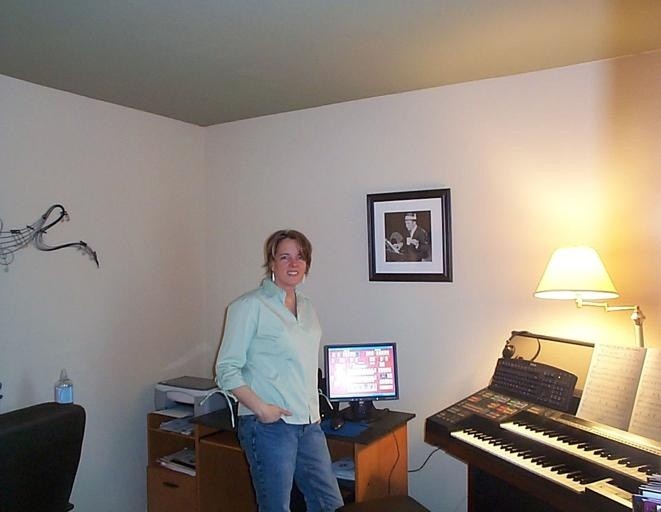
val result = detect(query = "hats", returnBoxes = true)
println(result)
[404,212,417,221]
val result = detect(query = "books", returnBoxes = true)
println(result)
[631,473,661,512]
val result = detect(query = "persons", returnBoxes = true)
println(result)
[394,212,430,262]
[213,230,347,512]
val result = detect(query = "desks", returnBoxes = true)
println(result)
[147,405,407,512]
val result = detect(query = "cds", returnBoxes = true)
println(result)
[334,460,354,470]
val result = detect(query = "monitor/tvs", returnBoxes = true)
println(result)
[323,342,400,422]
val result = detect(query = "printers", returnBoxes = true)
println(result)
[154,375,227,418]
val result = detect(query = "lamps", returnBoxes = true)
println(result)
[534,247,647,347]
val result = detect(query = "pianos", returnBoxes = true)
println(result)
[424,388,661,511]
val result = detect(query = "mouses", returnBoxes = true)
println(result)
[330,418,345,430]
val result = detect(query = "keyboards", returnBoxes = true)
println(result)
[490,358,578,412]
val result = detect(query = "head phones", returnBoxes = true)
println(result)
[502,330,540,361]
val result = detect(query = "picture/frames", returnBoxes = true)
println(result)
[365,188,453,283]
